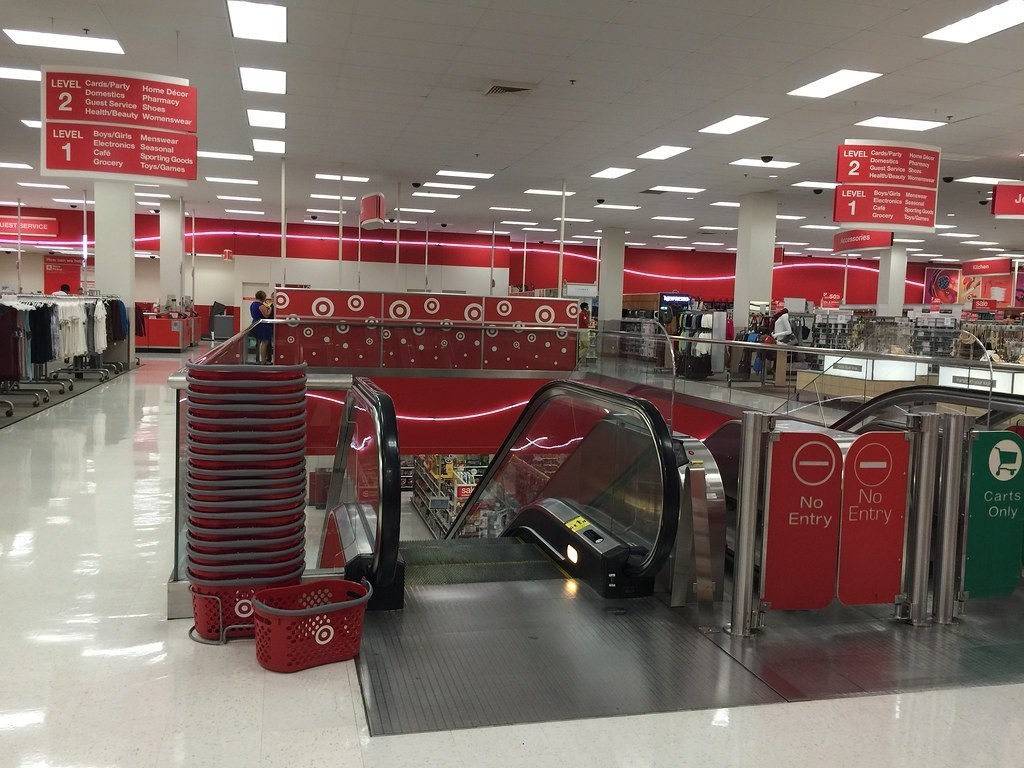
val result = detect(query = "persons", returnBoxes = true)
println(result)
[250,291,275,365]
[51,284,70,296]
[578,302,591,363]
[770,308,793,346]
[76,287,85,297]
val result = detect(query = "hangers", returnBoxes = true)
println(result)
[0,294,119,311]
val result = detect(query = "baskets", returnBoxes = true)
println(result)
[250,578,372,673]
[184,355,307,673]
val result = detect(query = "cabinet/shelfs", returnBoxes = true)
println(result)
[587,291,1024,402]
[400,455,488,539]
